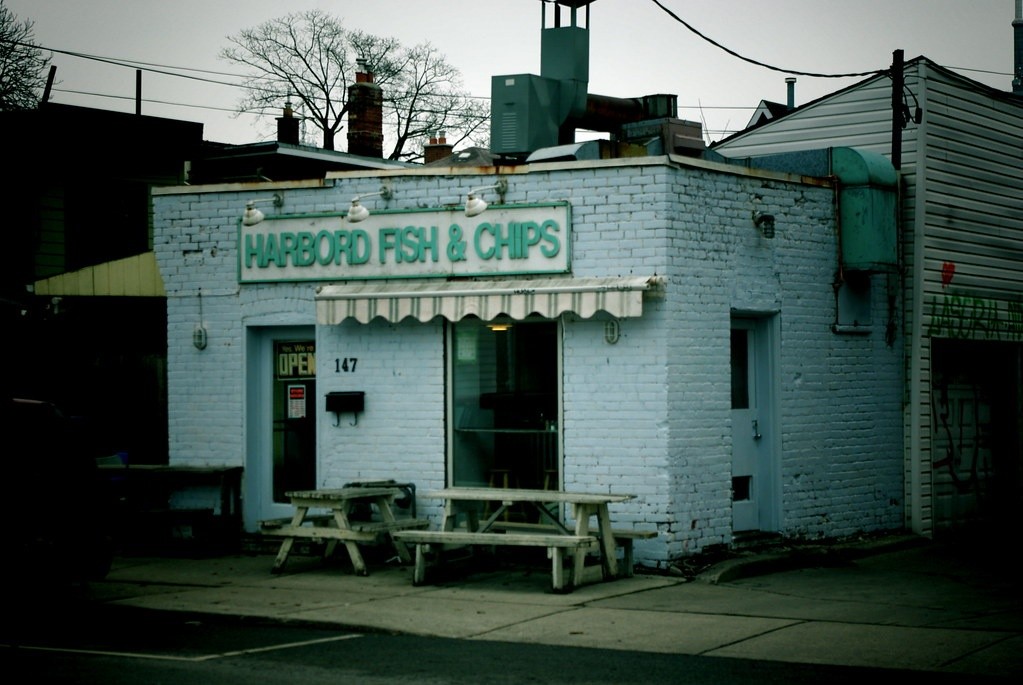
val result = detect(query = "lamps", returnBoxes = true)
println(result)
[193,327,206,349]
[347,186,391,223]
[243,193,284,225]
[753,210,776,238]
[487,324,511,331]
[464,180,508,218]
[605,320,619,344]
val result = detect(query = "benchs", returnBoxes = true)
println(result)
[258,513,334,529]
[353,517,430,533]
[459,520,657,540]
[391,530,597,548]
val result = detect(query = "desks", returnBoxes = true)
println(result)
[268,487,412,574]
[100,465,244,556]
[409,486,636,590]
